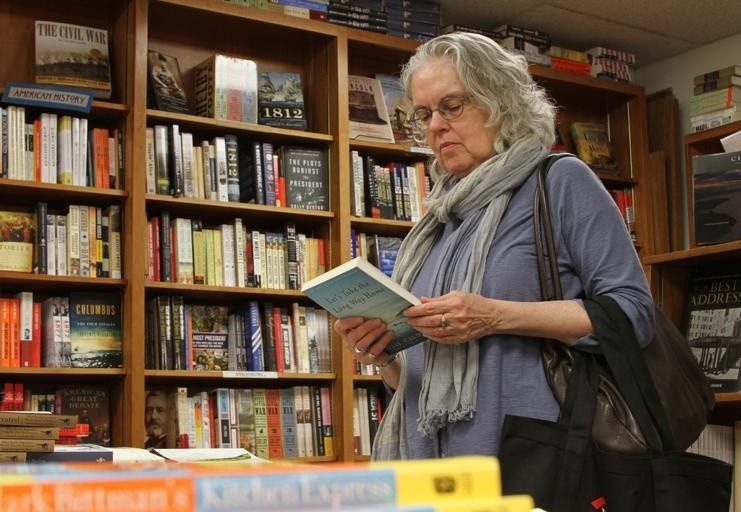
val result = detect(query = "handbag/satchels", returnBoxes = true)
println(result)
[539,305,715,455]
[500,414,733,512]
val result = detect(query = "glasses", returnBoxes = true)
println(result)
[407,93,472,132]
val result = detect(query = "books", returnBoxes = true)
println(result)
[348,73,433,454]
[144,48,335,456]
[269,0,442,41]
[677,64,741,463]
[1,410,534,511]
[300,254,430,354]
[1,19,125,444]
[551,122,637,242]
[443,24,634,81]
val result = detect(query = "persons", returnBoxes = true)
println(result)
[334,32,655,456]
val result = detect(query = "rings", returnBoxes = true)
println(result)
[441,315,446,328]
